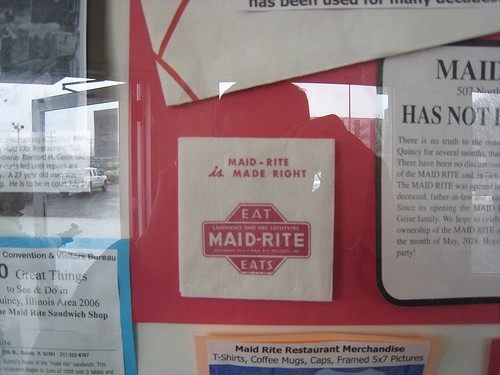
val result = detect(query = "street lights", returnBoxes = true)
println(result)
[12,121,24,180]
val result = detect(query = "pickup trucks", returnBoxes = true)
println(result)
[62,167,107,197]
